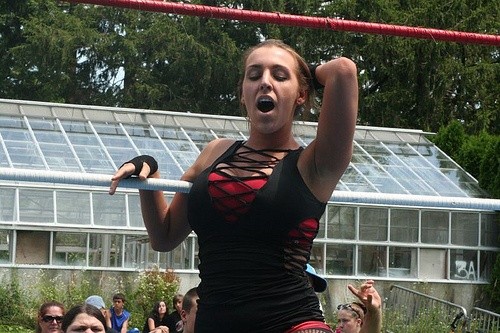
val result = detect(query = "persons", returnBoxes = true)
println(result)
[338,301,366,333]
[306,263,382,333]
[32,287,198,333]
[109,39,358,333]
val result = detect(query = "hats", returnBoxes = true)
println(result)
[85,295,105,309]
[305,263,327,293]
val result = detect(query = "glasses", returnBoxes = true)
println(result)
[337,304,360,318]
[40,316,65,323]
